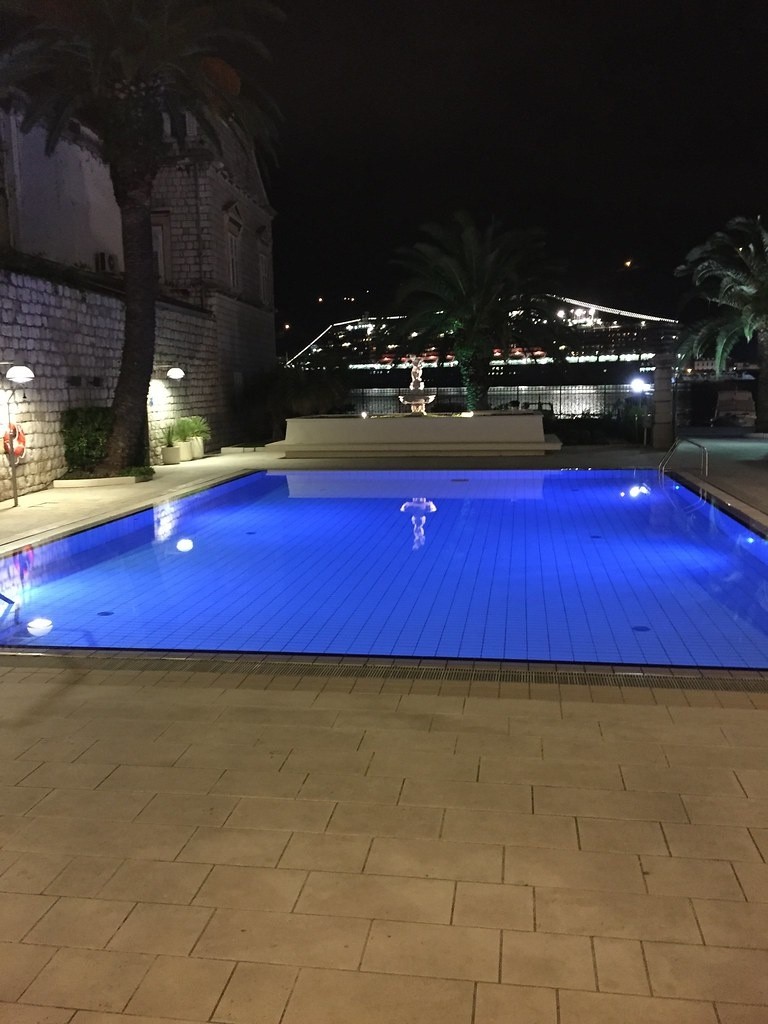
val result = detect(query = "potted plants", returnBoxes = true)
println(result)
[161,415,212,464]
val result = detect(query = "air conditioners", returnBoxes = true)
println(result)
[98,251,119,273]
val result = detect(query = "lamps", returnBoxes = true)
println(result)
[152,364,185,379]
[0,362,35,383]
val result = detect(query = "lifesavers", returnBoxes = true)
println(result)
[13,545,35,575]
[4,424,26,456]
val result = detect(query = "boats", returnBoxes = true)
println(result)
[521,401,606,446]
